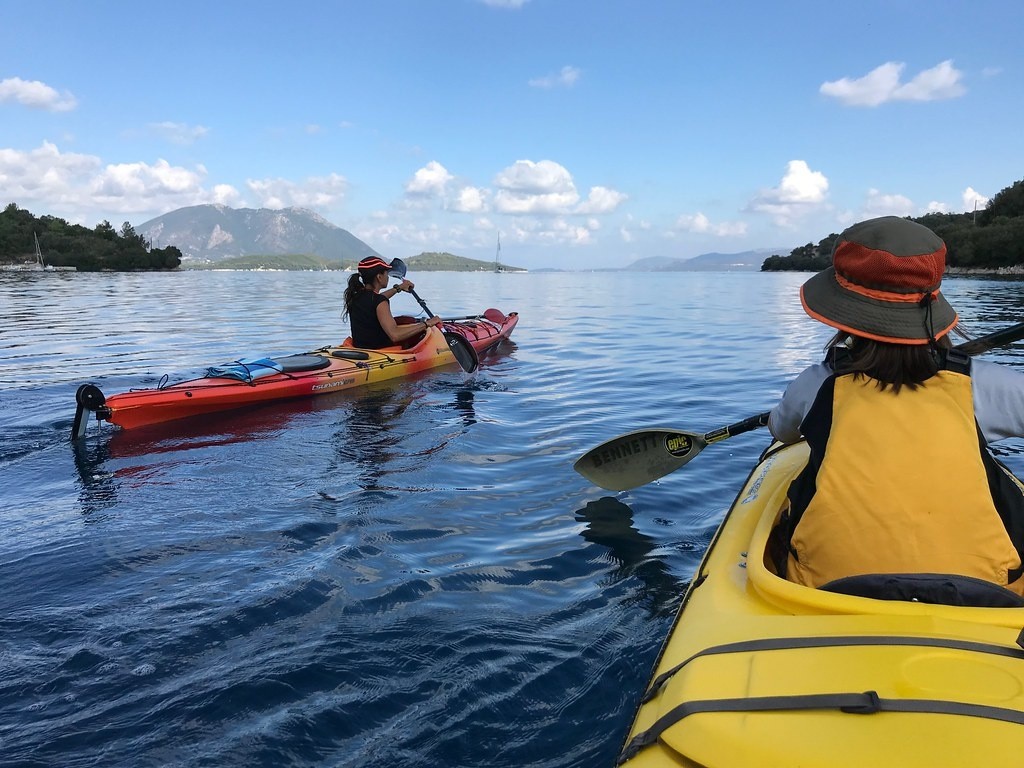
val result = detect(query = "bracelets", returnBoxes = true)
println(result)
[423,321,428,330]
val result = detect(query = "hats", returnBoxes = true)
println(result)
[799,216,959,345]
[357,256,392,269]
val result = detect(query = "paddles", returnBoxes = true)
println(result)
[388,257,480,374]
[572,322,1024,493]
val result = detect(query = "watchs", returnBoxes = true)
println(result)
[393,283,401,293]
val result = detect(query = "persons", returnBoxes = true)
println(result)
[340,256,442,347]
[765,216,1023,607]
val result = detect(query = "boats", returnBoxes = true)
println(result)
[69,306,520,443]
